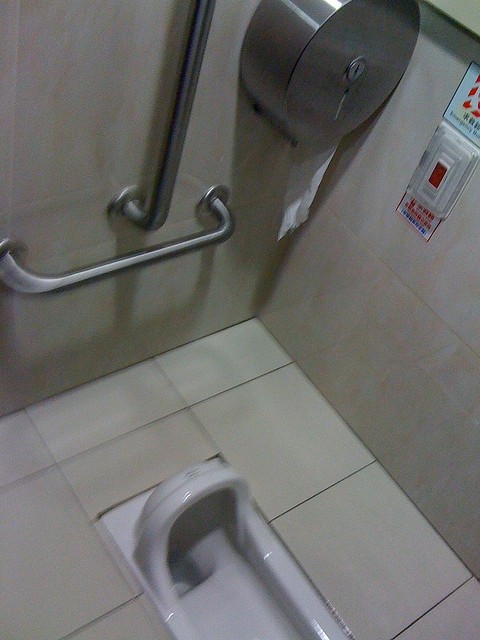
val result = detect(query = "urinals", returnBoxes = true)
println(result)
[99,455,354,640]
